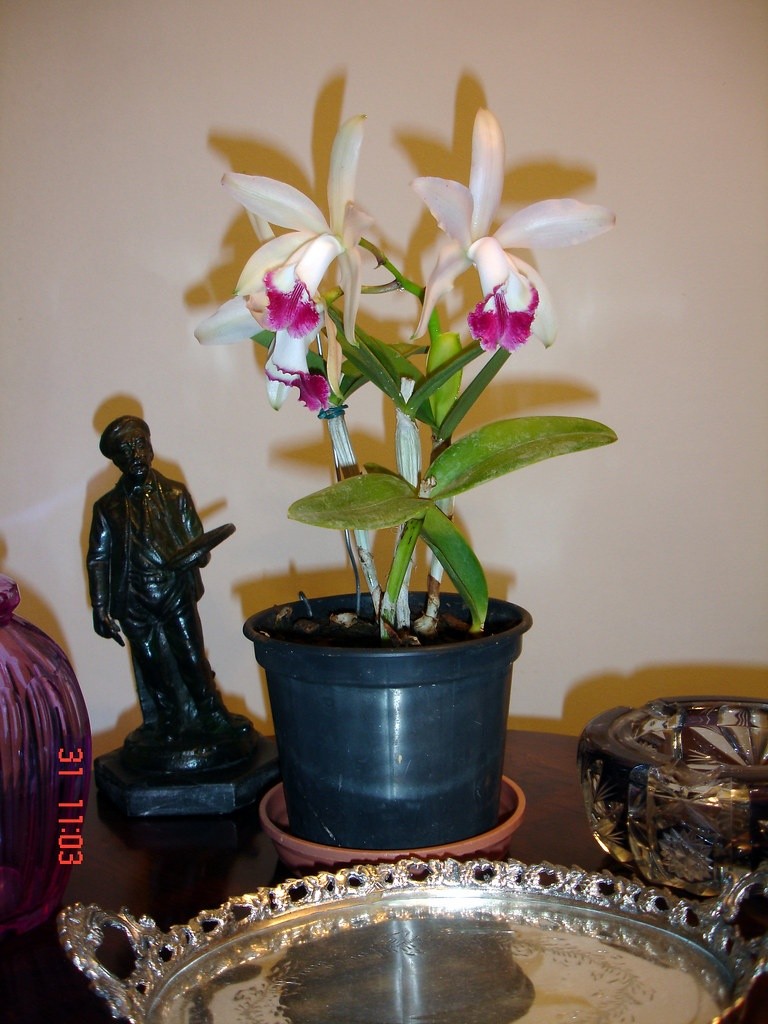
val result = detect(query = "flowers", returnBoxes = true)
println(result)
[192,107,619,637]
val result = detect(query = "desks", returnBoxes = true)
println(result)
[1,722,625,1023]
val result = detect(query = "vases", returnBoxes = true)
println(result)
[242,590,534,862]
